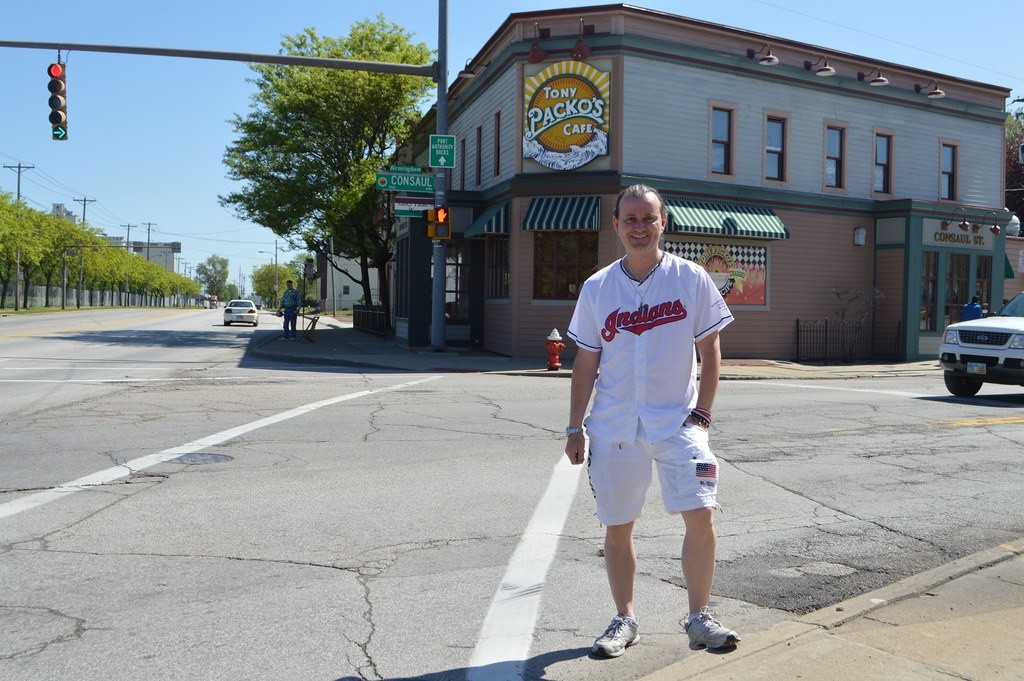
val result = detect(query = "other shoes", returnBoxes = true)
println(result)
[280,338,288,342]
[289,337,296,341]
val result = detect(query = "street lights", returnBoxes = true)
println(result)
[258,250,279,310]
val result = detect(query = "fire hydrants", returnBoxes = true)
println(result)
[545,328,567,369]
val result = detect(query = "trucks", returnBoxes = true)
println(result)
[250,296,262,309]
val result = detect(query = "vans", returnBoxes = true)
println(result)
[210,300,218,309]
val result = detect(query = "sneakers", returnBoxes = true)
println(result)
[591,613,641,657]
[679,605,742,650]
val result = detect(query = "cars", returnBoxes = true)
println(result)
[939,290,1024,398]
[224,299,261,325]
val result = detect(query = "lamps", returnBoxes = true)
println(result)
[914,79,945,99]
[431,95,459,109]
[972,212,1000,236]
[458,58,490,78]
[570,18,594,62]
[857,66,889,86]
[941,208,969,232]
[528,21,550,63]
[747,43,780,66]
[803,54,836,77]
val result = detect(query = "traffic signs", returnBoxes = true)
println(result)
[429,135,456,168]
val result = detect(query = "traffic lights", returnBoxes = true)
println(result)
[432,207,450,239]
[48,63,67,140]
[422,209,434,238]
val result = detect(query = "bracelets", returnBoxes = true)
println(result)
[690,407,711,428]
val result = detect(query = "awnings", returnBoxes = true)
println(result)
[658,195,791,239]
[464,201,509,238]
[522,197,601,231]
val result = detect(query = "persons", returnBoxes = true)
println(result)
[279,280,302,342]
[960,296,983,322]
[565,184,741,659]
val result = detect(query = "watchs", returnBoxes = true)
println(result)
[566,427,583,437]
